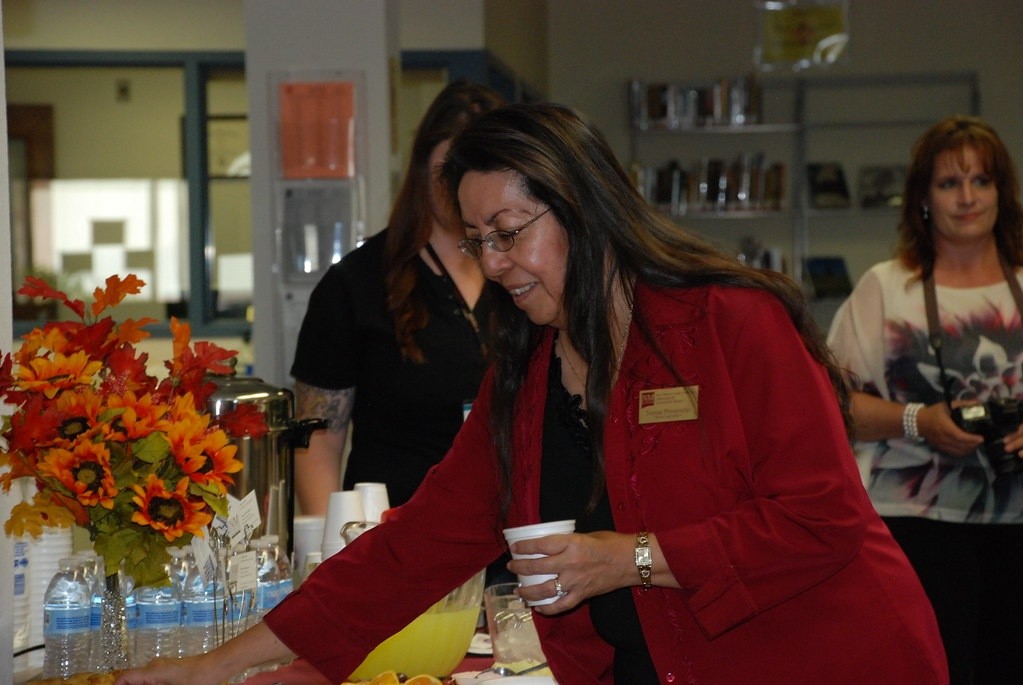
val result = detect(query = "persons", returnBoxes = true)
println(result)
[290,74,544,516]
[115,100,952,685]
[823,112,1023,684]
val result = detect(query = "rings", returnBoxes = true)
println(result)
[555,578,565,597]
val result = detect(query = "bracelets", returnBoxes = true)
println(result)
[903,401,926,437]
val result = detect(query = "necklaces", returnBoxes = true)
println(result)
[557,300,631,389]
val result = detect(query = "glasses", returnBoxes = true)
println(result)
[457,208,551,259]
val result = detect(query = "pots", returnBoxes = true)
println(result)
[156,369,328,557]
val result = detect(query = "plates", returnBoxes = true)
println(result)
[466,641,494,656]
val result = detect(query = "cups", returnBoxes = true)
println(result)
[295,470,389,568]
[503,517,578,606]
[485,579,532,664]
[0,420,73,682]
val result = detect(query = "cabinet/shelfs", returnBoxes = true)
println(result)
[628,69,1004,349]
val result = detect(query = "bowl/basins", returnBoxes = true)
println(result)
[312,546,487,679]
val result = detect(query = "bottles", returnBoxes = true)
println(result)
[41,536,298,684]
[623,66,795,211]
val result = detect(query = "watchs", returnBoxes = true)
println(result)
[635,531,652,588]
